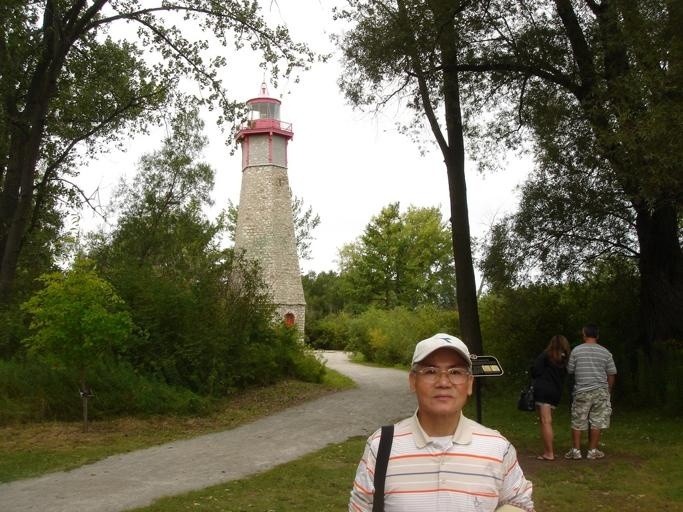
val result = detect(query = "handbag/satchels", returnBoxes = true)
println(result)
[517,386,535,411]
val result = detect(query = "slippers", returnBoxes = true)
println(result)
[535,455,555,461]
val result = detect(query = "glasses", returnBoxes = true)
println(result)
[411,364,472,386]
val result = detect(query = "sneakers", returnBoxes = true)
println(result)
[586,448,606,460]
[564,448,583,460]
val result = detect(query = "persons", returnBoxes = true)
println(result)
[563,323,618,460]
[346,332,538,512]
[530,334,571,461]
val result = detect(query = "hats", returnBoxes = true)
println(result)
[410,331,475,368]
[583,323,600,337]
